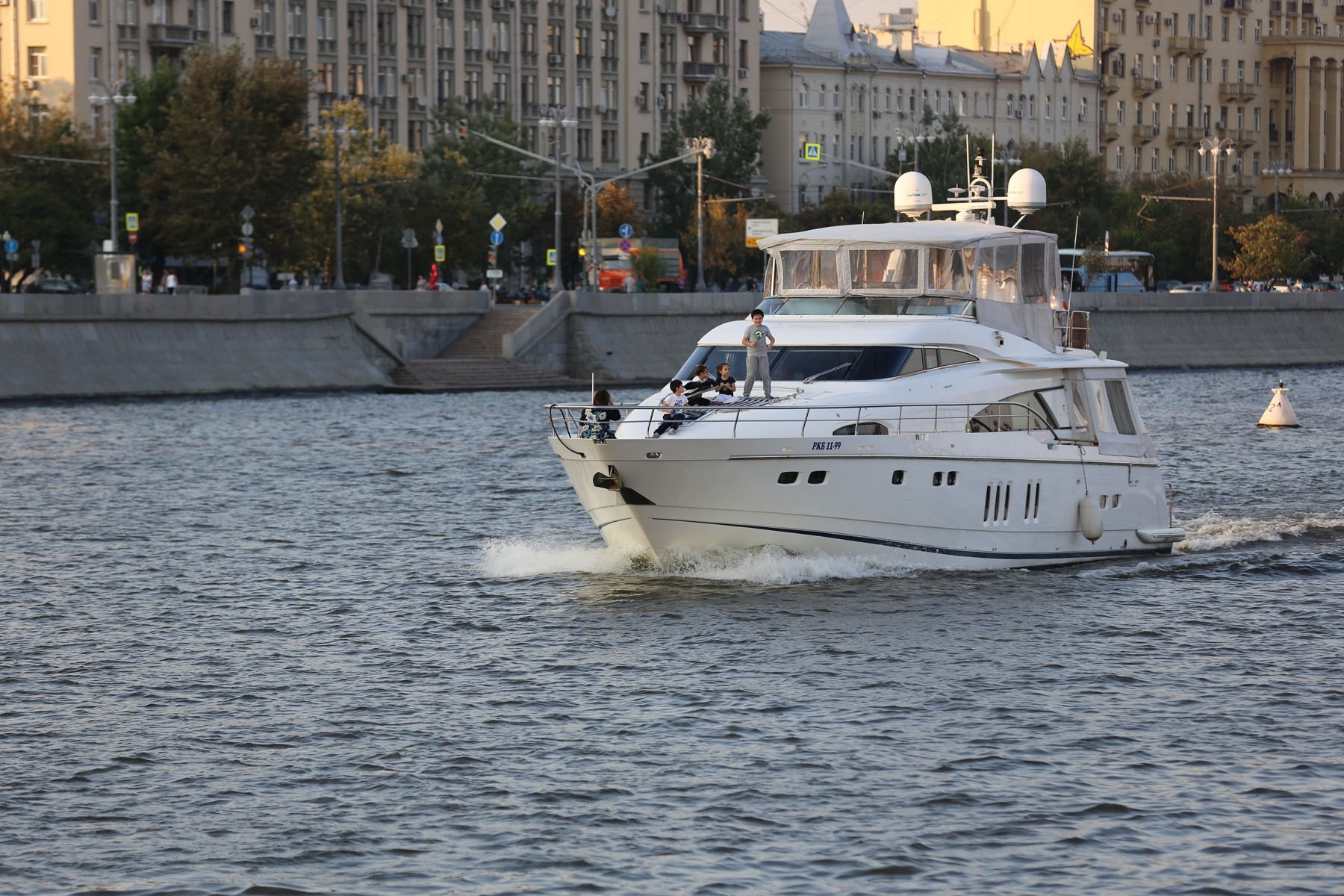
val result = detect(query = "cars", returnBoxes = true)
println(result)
[0,261,88,294]
[1157,275,1344,293]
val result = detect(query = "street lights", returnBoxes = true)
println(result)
[1196,133,1236,289]
[683,134,716,291]
[1260,157,1293,216]
[895,122,937,171]
[85,76,137,255]
[320,116,358,289]
[990,143,1022,228]
[537,108,578,297]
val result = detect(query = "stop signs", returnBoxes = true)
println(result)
[128,230,137,244]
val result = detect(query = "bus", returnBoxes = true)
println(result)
[1050,247,1159,297]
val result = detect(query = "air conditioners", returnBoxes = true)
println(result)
[873,111,882,118]
[1016,109,1023,119]
[739,69,748,77]
[836,112,846,119]
[1078,113,1084,120]
[900,112,908,119]
[1111,13,1323,171]
[254,5,688,115]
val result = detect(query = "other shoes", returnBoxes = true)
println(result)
[766,396,774,400]
[743,396,749,401]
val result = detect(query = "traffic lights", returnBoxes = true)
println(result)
[237,236,249,261]
[457,118,470,143]
[245,236,254,260]
[487,243,497,270]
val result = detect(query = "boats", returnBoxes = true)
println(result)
[541,122,1194,571]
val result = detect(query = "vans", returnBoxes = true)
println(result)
[596,246,685,293]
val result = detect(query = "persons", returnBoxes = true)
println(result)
[142,267,178,296]
[1237,278,1303,292]
[741,309,776,402]
[579,389,622,439]
[686,365,714,406]
[651,379,693,439]
[448,277,604,306]
[416,275,427,291]
[712,362,741,404]
[1061,273,1083,292]
[622,269,758,292]
